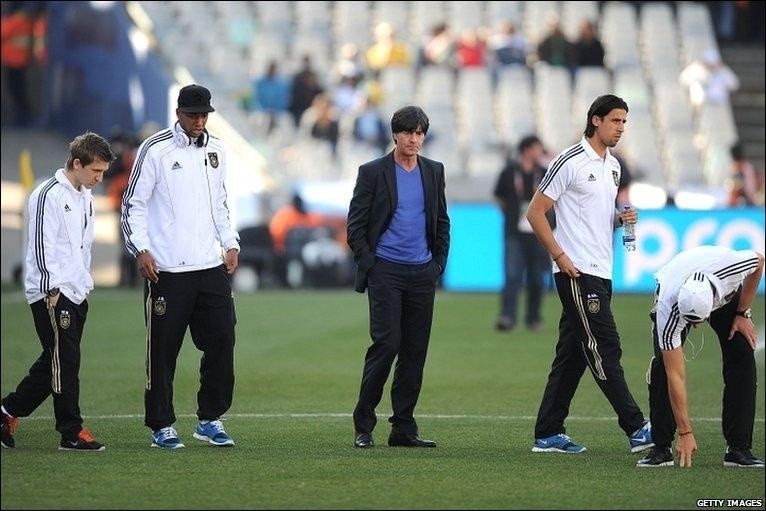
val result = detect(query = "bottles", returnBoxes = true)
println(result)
[622,205,637,253]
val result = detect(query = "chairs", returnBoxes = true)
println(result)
[139,0,737,209]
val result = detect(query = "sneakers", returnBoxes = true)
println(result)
[192,419,235,446]
[724,445,764,467]
[57,428,105,451]
[626,420,654,453]
[150,425,185,450]
[635,447,674,467]
[0,404,16,448]
[531,433,587,453]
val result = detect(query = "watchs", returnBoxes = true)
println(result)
[737,308,752,318]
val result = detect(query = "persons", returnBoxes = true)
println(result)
[635,244,765,469]
[253,13,739,161]
[120,84,242,449]
[527,95,655,453]
[0,133,117,452]
[493,134,555,330]
[345,106,450,450]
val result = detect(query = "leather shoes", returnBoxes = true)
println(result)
[354,432,374,447]
[387,435,437,447]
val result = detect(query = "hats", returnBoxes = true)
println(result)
[178,85,215,113]
[678,272,713,323]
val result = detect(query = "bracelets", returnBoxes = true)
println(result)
[548,251,566,261]
[678,430,692,436]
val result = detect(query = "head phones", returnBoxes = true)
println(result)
[170,120,209,148]
